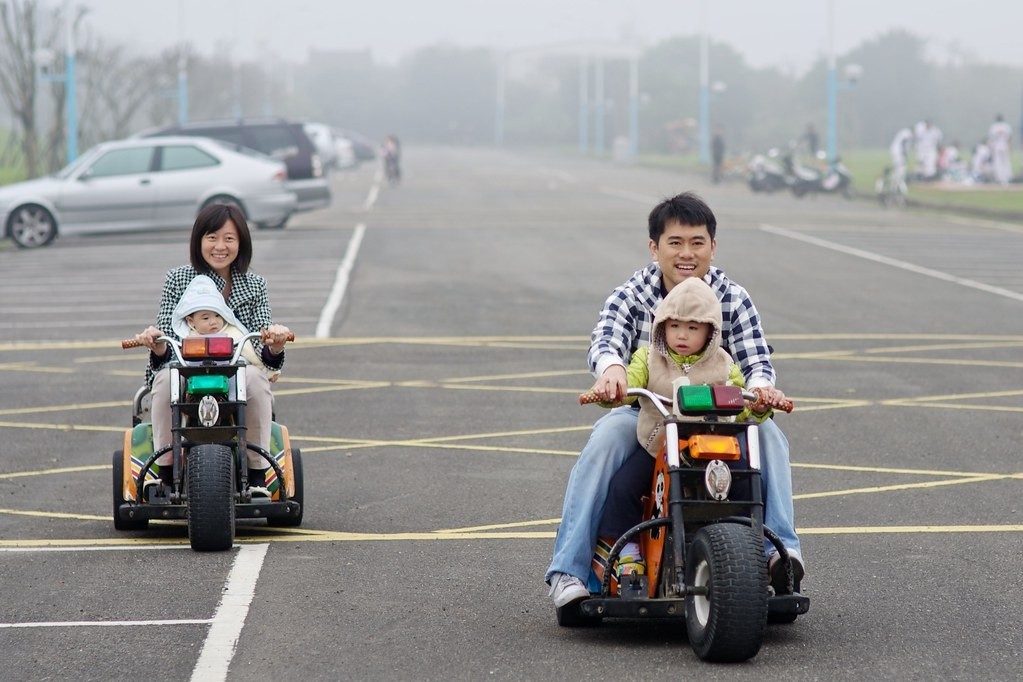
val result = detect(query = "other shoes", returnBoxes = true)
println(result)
[247,487,271,498]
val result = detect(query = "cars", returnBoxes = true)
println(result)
[126,117,377,181]
[219,140,288,178]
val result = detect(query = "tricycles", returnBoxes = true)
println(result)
[556,382,811,665]
[111,327,306,552]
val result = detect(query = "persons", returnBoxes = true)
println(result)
[590,276,782,595]
[172,275,280,383]
[381,133,403,184]
[544,190,805,610]
[713,128,724,181]
[136,205,289,500]
[890,112,1013,192]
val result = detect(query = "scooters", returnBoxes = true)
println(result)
[747,152,863,201]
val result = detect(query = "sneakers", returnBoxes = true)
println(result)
[548,572,592,609]
[612,556,646,598]
[769,548,805,594]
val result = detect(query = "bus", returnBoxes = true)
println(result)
[0,137,298,249]
